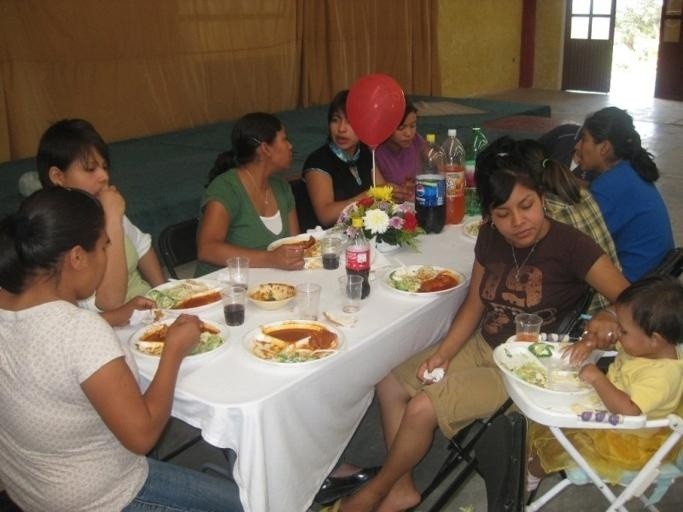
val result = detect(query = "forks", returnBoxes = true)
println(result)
[296,348,350,356]
[166,278,208,292]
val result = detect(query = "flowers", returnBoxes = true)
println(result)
[344,188,424,254]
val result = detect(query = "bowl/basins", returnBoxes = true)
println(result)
[248,282,295,309]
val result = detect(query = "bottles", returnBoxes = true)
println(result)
[414,134,445,234]
[440,128,465,222]
[345,218,370,299]
[464,125,489,216]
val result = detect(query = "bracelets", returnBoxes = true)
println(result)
[606,307,617,315]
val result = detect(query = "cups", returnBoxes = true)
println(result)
[514,312,543,341]
[320,236,341,271]
[226,255,249,290]
[294,283,321,321]
[219,286,247,327]
[338,274,365,314]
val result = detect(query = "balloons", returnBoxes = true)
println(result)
[344,72,406,150]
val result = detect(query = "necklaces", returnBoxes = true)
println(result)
[242,165,270,207]
[511,241,536,279]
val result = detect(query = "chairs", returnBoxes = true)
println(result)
[403,284,596,512]
[158,218,197,278]
[493,331,683,512]
[645,247,682,281]
[288,179,324,234]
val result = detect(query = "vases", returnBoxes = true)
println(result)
[374,239,401,252]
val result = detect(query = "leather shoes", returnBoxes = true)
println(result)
[315,467,381,504]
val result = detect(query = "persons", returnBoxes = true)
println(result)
[301,91,416,231]
[526,272,683,491]
[192,112,306,279]
[376,101,431,184]
[36,120,383,504]
[0,186,245,512]
[519,137,623,316]
[326,156,630,512]
[576,107,674,282]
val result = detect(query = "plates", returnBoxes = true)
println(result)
[492,341,597,396]
[461,220,483,241]
[143,278,221,313]
[382,264,468,295]
[265,232,346,260]
[128,318,230,358]
[242,320,346,364]
